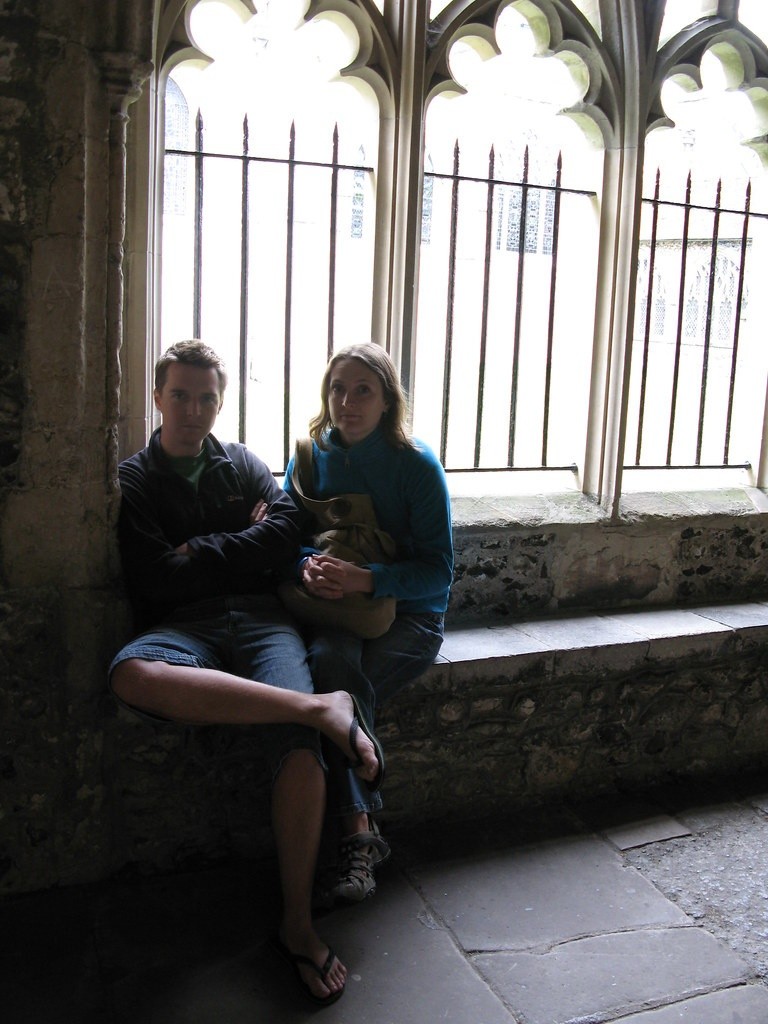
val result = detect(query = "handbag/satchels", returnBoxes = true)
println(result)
[285,494,396,638]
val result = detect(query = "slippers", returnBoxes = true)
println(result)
[278,930,347,1007]
[342,692,385,794]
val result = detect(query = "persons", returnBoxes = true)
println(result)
[108,339,386,1007]
[285,343,453,908]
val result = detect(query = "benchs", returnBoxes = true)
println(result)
[379,597,768,829]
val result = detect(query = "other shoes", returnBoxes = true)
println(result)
[332,815,391,903]
